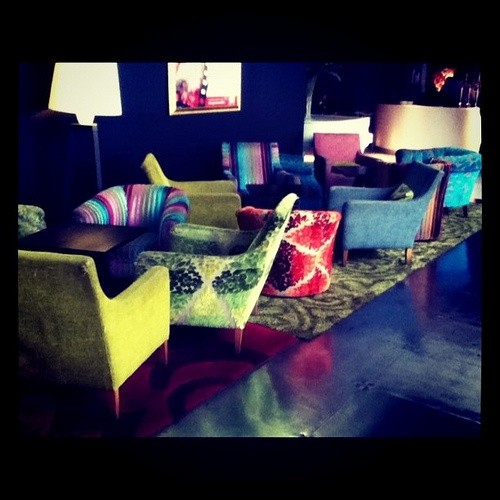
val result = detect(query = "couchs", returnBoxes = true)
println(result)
[222,141,301,209]
[313,133,382,186]
[73,183,190,277]
[235,205,342,298]
[18,250,171,421]
[395,146,482,218]
[135,193,300,361]
[143,153,241,228]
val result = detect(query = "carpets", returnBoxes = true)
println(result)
[328,160,445,267]
[249,203,482,340]
[74,323,296,437]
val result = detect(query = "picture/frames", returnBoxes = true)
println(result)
[168,62,241,116]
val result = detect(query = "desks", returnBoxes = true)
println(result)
[355,152,397,187]
[19,222,142,254]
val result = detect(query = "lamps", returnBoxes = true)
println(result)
[48,63,122,125]
